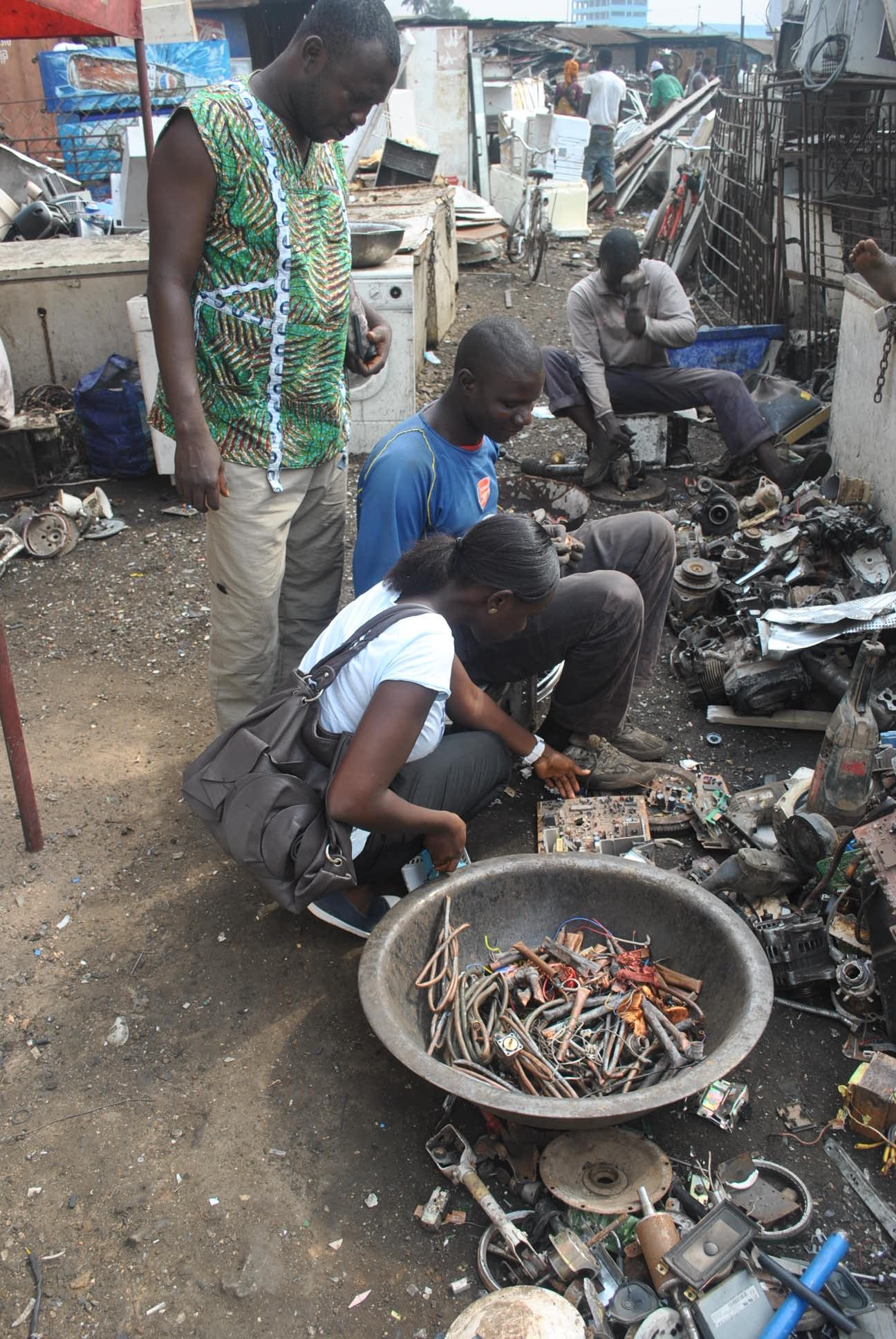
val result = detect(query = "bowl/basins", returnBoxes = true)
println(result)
[348,220,412,268]
[358,853,774,1131]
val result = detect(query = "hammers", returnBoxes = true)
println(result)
[621,270,646,307]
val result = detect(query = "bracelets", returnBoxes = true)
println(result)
[519,734,546,766]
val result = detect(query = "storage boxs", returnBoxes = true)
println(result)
[484,79,594,237]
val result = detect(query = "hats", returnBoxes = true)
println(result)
[649,60,663,73]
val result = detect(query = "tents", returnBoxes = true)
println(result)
[0,0,154,176]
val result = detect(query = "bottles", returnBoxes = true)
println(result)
[67,53,208,98]
[78,126,124,152]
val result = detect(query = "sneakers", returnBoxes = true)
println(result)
[607,724,668,761]
[558,732,655,791]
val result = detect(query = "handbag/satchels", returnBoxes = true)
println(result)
[180,600,428,914]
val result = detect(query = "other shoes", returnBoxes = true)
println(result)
[779,450,833,499]
[605,206,617,219]
[582,454,609,487]
[303,896,388,939]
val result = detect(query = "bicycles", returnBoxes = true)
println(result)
[499,129,559,284]
[648,135,726,267]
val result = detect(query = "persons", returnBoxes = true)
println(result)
[293,512,591,939]
[849,238,896,305]
[144,0,400,741]
[581,48,626,222]
[647,61,684,121]
[682,50,704,91]
[554,61,583,114]
[688,57,724,127]
[352,320,679,791]
[540,227,833,497]
